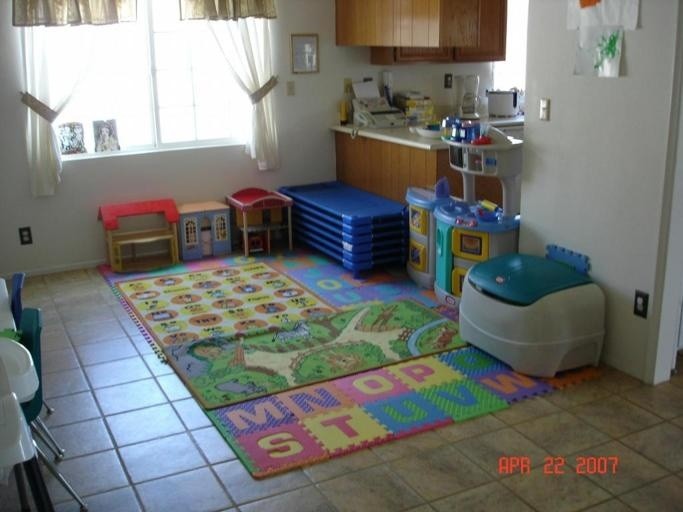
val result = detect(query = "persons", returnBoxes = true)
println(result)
[96,121,119,152]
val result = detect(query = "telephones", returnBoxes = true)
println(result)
[352,111,374,130]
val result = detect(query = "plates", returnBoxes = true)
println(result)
[414,127,441,139]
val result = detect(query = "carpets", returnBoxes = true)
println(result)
[95,254,609,480]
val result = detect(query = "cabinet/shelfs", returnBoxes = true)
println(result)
[335,0,482,49]
[370,0,507,65]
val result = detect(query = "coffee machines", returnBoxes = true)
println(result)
[453,73,479,120]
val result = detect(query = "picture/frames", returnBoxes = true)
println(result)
[289,32,319,75]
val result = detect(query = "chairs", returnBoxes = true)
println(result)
[10,272,64,462]
[14,308,89,512]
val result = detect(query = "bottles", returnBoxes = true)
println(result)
[463,147,495,173]
[441,112,479,144]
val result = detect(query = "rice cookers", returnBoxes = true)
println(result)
[486,90,519,117]
